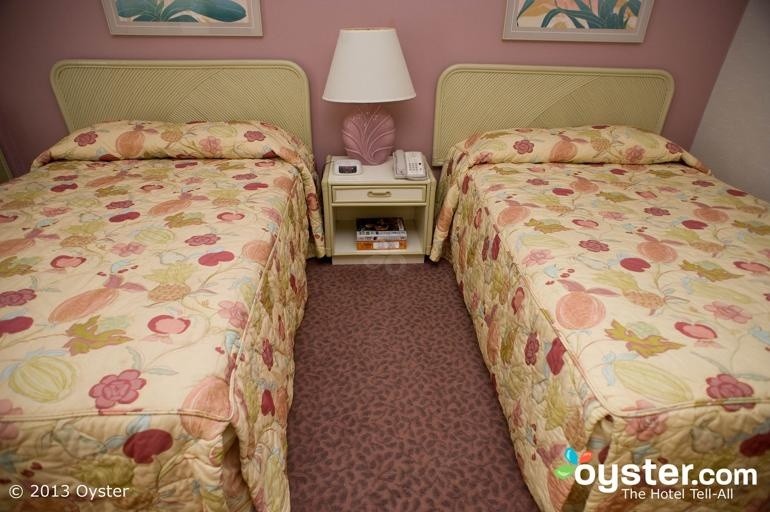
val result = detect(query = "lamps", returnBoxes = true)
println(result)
[321,26,417,166]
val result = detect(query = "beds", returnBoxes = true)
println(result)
[0,55,323,511]
[431,64,770,512]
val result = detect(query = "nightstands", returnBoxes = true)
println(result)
[320,155,437,264]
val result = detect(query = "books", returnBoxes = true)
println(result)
[355,240,410,249]
[357,215,408,241]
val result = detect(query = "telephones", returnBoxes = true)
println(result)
[392,149,426,179]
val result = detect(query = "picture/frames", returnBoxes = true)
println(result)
[502,0,655,44]
[102,0,263,37]
